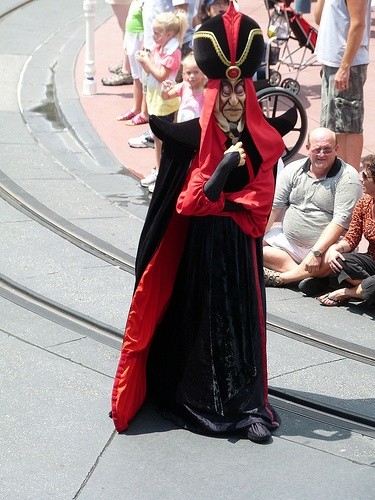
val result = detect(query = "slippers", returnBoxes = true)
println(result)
[117,112,136,121]
[125,113,149,126]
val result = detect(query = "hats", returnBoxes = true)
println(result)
[205,0,237,12]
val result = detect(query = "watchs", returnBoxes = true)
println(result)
[310,248,322,257]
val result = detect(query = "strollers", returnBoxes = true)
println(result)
[264,0,318,95]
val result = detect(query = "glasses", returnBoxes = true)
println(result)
[308,142,338,154]
[361,171,375,182]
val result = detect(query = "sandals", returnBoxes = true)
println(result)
[316,291,350,306]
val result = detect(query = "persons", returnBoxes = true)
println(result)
[311,0,372,173]
[115,0,149,127]
[160,51,210,124]
[101,0,134,86]
[191,0,235,51]
[136,9,189,194]
[262,128,364,296]
[128,0,196,148]
[316,153,375,306]
[108,0,286,443]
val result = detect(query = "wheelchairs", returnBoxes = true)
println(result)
[254,36,309,162]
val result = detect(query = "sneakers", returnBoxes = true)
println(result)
[139,170,159,192]
[107,63,123,74]
[127,131,155,149]
[101,70,133,86]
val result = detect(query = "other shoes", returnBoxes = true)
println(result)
[298,277,343,297]
[247,422,272,442]
[262,266,283,287]
